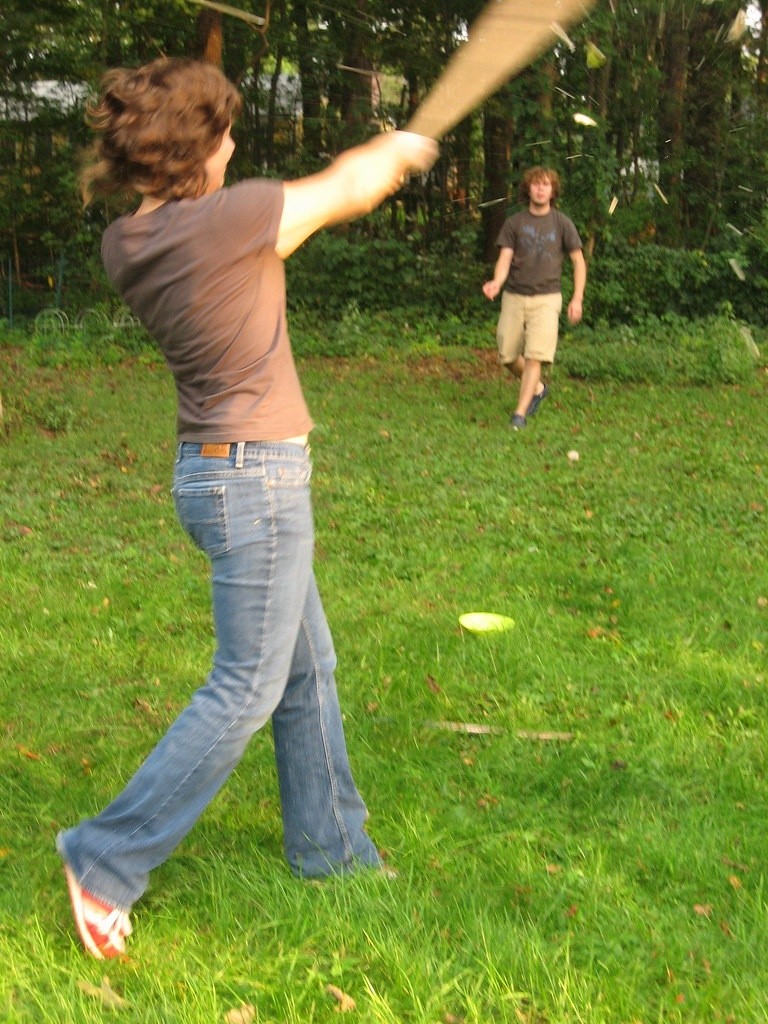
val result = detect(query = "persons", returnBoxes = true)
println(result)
[55,58,440,962]
[482,167,586,430]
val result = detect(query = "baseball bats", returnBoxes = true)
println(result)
[400,0,611,156]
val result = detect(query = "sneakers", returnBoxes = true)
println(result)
[304,865,401,888]
[54,828,133,961]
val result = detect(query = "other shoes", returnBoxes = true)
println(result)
[527,385,548,416]
[509,414,526,430]
